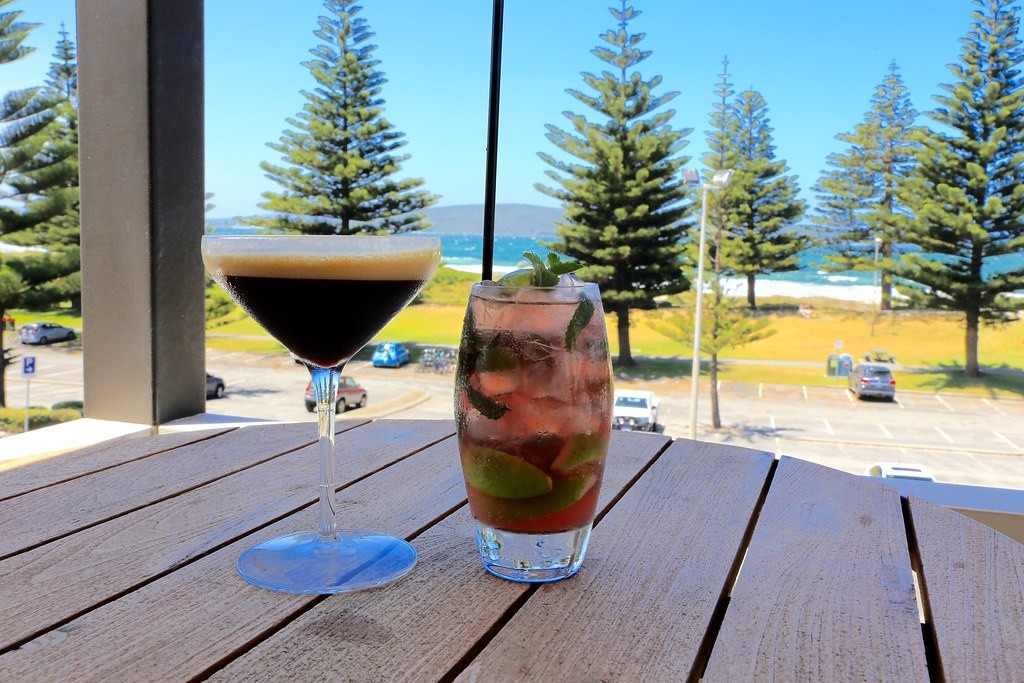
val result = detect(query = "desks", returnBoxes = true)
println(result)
[0,420,1024,683]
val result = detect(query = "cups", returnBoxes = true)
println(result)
[454,282,614,584]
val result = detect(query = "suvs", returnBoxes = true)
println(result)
[305,376,367,416]
[848,365,895,401]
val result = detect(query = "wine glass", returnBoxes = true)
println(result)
[202,233,444,594]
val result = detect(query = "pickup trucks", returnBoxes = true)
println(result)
[612,390,659,434]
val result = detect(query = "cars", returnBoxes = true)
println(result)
[206,374,225,397]
[21,323,75,344]
[372,342,410,368]
[866,462,934,483]
